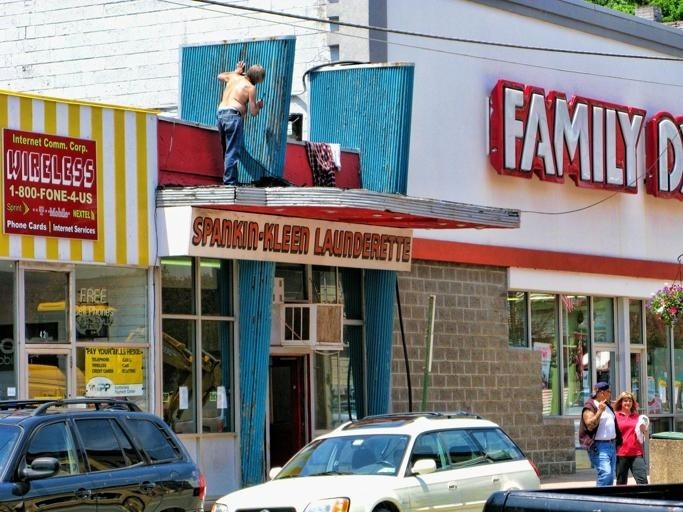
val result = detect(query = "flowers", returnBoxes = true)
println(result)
[642,281,682,328]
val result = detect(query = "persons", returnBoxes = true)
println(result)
[578,381,617,485]
[216,60,269,184]
[610,391,650,485]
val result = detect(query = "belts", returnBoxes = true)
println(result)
[596,439,617,443]
[219,109,241,116]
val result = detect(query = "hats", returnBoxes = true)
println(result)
[595,382,608,391]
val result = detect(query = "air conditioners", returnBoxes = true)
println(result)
[269,300,344,351]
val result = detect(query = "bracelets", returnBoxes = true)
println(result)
[642,431,647,434]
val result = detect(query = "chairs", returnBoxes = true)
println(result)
[351,445,473,473]
